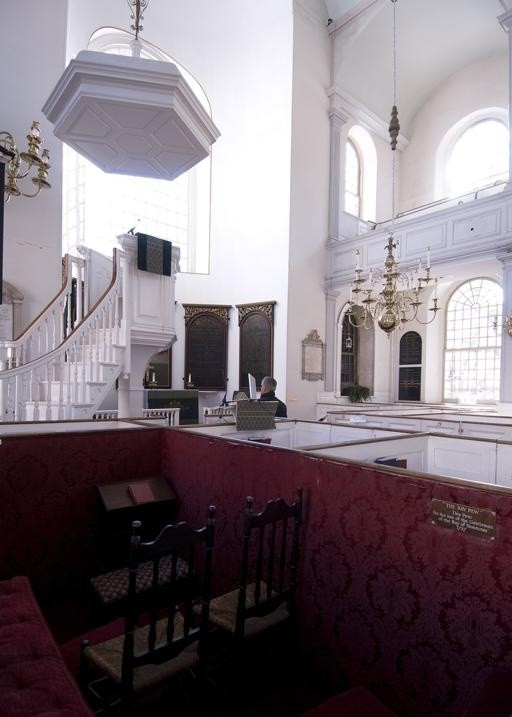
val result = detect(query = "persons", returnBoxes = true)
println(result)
[257,375,287,417]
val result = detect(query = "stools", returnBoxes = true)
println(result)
[88,553,197,627]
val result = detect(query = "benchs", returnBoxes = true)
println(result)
[0,575,96,717]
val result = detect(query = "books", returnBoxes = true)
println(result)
[248,435,272,444]
[128,483,157,504]
[395,456,408,469]
[374,455,396,465]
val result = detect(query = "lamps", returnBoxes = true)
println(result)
[0,121,53,204]
[345,0,440,334]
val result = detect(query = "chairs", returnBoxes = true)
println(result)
[78,483,311,717]
[297,650,512,717]
